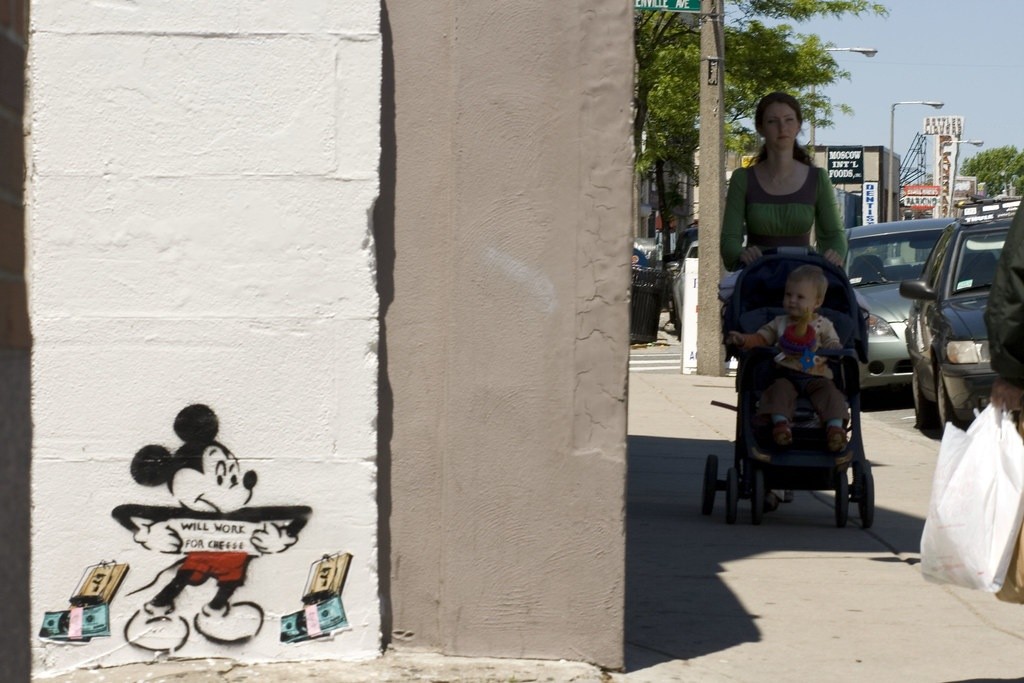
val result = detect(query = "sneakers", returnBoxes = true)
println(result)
[827,426,852,464]
[774,420,792,445]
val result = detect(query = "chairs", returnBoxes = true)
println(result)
[852,255,924,283]
[956,251,998,289]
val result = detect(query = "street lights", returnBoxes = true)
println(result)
[940,140,983,218]
[886,98,944,221]
[811,48,877,161]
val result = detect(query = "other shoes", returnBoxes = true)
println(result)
[778,489,793,504]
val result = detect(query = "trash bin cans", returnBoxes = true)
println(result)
[629,266,672,343]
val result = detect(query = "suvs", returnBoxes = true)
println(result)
[899,189,1023,434]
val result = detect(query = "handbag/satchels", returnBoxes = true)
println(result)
[921,395,1024,605]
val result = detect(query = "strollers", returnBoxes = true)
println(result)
[701,248,875,529]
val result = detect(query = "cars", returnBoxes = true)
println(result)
[668,225,699,332]
[842,219,956,410]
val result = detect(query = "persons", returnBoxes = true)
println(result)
[720,92,848,512]
[984,194,1024,429]
[726,264,849,452]
